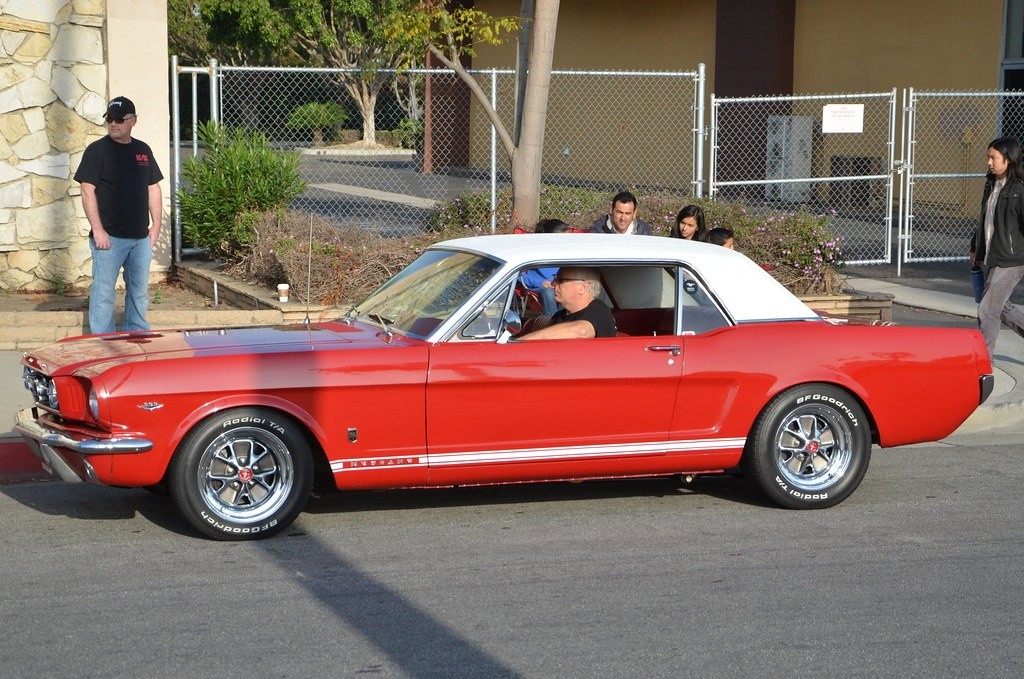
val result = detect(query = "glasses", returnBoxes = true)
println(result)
[551,273,585,285]
[105,116,134,124]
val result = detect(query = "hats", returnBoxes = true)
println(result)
[103,96,136,119]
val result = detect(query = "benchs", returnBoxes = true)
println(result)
[610,307,725,335]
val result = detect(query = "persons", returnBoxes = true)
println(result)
[671,205,708,243]
[73,97,164,335]
[970,137,1023,370]
[518,219,571,318]
[514,267,617,340]
[589,191,654,235]
[708,228,735,250]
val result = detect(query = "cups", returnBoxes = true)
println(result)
[971,266,985,303]
[277,284,289,302]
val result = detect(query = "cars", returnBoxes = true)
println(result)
[12,232,993,542]
